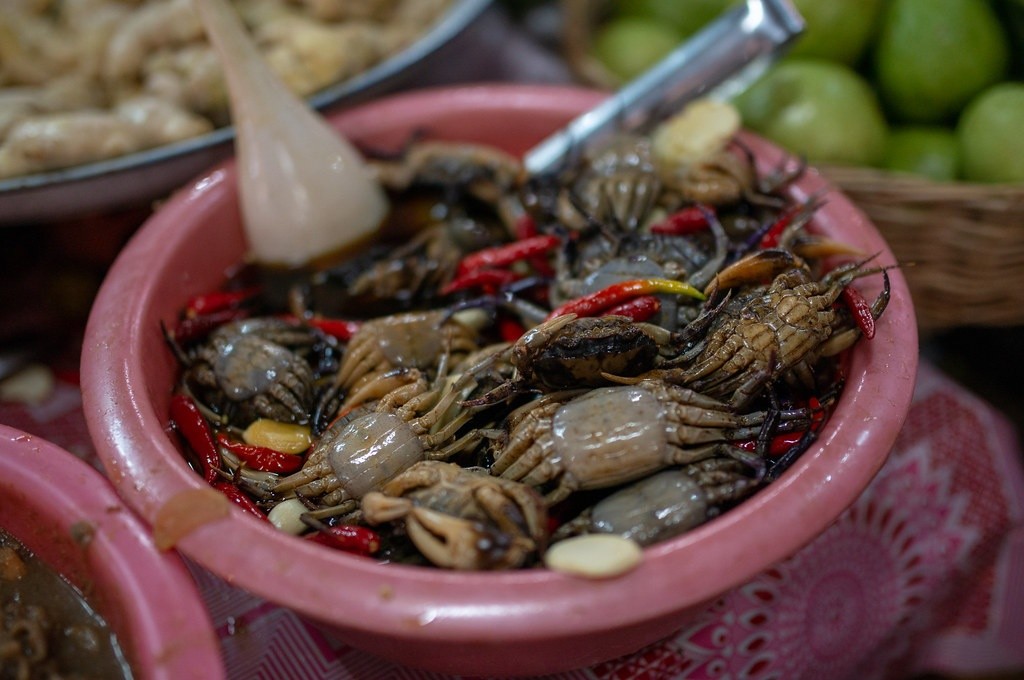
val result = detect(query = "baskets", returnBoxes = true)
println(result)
[819,163,1023,328]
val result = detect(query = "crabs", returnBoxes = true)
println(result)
[159,135,918,576]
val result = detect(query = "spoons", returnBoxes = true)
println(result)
[203,0,389,266]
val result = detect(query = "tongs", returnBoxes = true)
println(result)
[527,1,808,181]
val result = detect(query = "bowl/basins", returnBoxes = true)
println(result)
[2,427,225,679]
[80,82,921,677]
[1,1,488,224]
